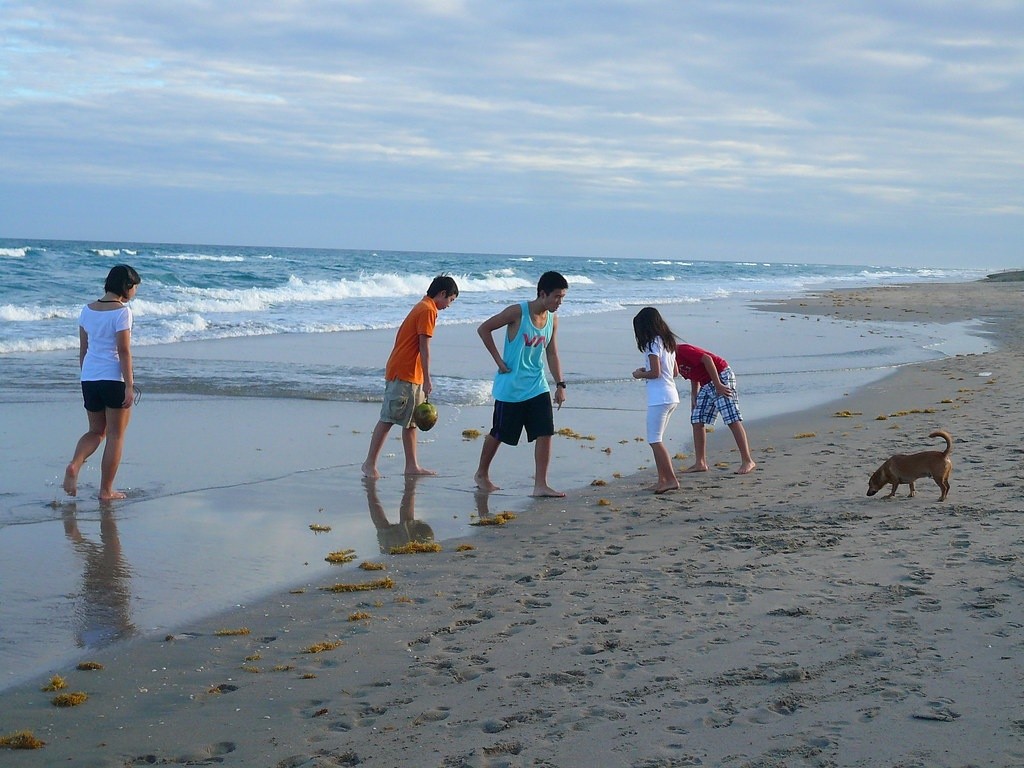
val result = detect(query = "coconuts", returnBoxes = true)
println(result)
[414,397,438,431]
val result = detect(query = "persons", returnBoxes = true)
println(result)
[670,342,757,475]
[357,470,438,552]
[631,306,684,495]
[59,499,141,652]
[361,269,461,478]
[472,271,570,498]
[61,263,141,500]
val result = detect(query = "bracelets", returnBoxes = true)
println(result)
[556,382,566,389]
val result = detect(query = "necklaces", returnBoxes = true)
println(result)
[98,298,124,306]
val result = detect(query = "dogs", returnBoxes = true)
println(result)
[866,430,953,501]
